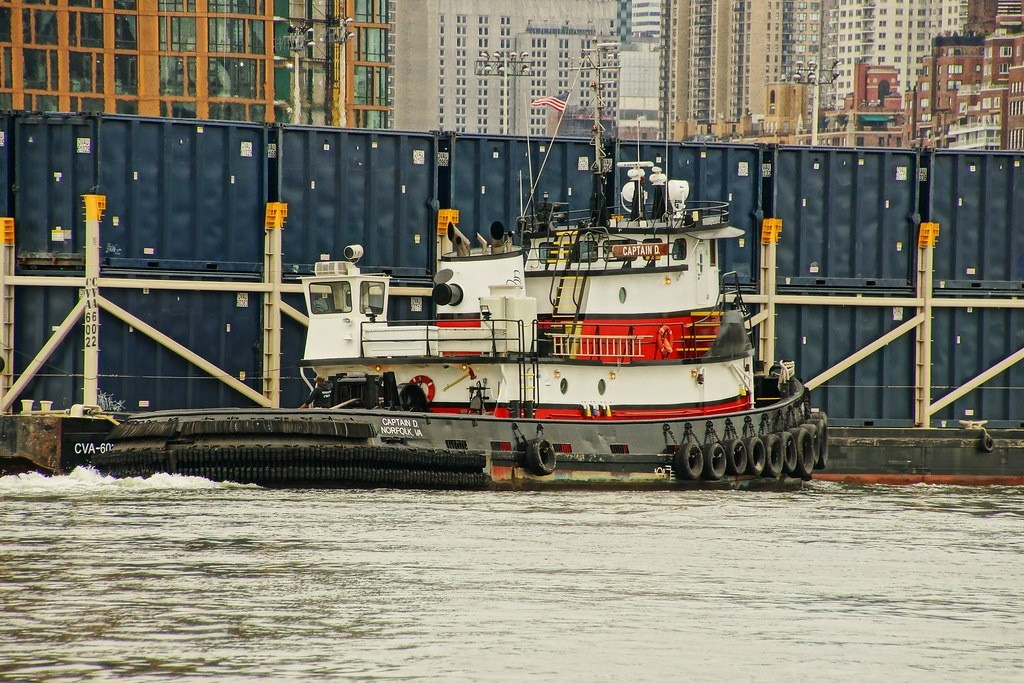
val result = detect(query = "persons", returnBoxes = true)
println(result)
[300,374,334,409]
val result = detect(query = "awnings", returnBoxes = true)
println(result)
[861,116,889,123]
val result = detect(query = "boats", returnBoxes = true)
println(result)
[86,15,828,492]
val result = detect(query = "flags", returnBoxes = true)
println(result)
[529,93,569,111]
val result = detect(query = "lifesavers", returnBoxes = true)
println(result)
[674,411,829,481]
[983,436,994,453]
[409,375,435,402]
[524,438,557,475]
[656,326,674,356]
